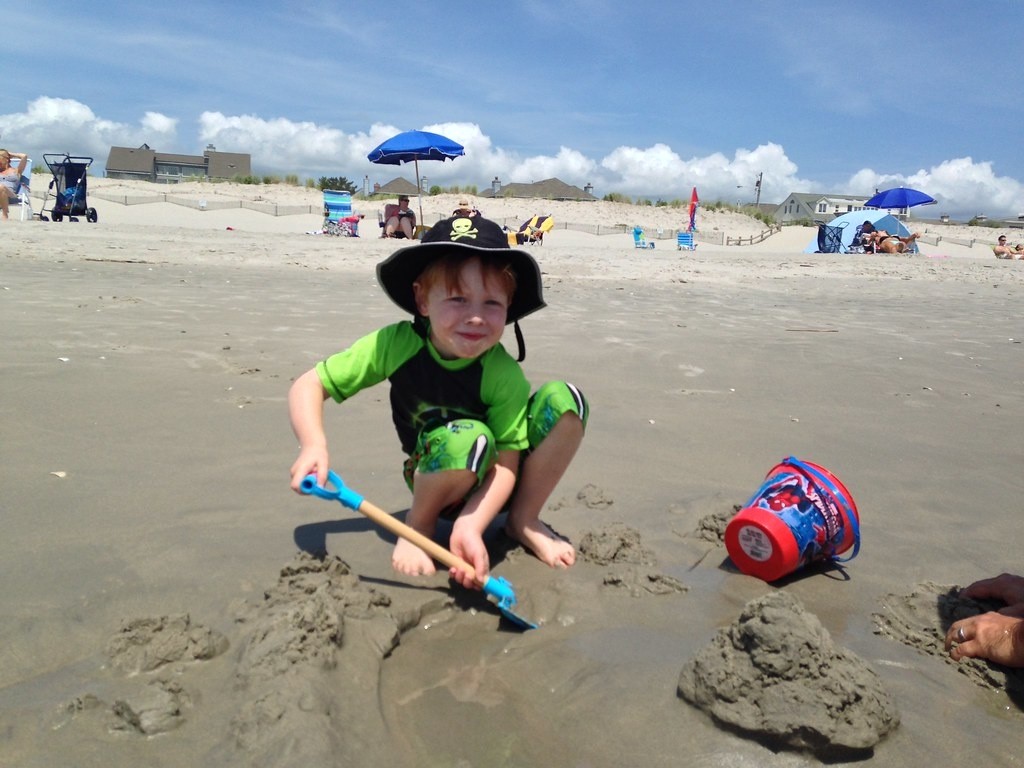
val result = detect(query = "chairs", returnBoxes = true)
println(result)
[633,228,656,248]
[322,189,364,237]
[8,158,32,219]
[991,245,1018,259]
[379,203,405,238]
[503,213,552,246]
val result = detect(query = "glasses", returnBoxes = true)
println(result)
[1001,240,1006,241]
[459,203,468,206]
[402,199,409,202]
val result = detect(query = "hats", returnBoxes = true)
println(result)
[376,216,548,326]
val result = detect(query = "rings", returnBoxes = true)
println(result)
[958,626,966,641]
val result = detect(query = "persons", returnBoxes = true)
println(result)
[868,227,921,254]
[384,195,417,241]
[0,148,28,221]
[285,214,590,592]
[994,234,1024,260]
[945,573,1024,668]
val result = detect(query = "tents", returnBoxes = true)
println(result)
[802,209,920,254]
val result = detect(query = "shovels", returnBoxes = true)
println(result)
[299,469,541,631]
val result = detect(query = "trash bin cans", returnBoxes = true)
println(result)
[817,225,844,253]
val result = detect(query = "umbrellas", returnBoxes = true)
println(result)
[862,187,936,245]
[367,128,465,242]
[689,187,698,232]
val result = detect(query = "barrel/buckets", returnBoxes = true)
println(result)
[678,233,693,249]
[724,456,861,582]
